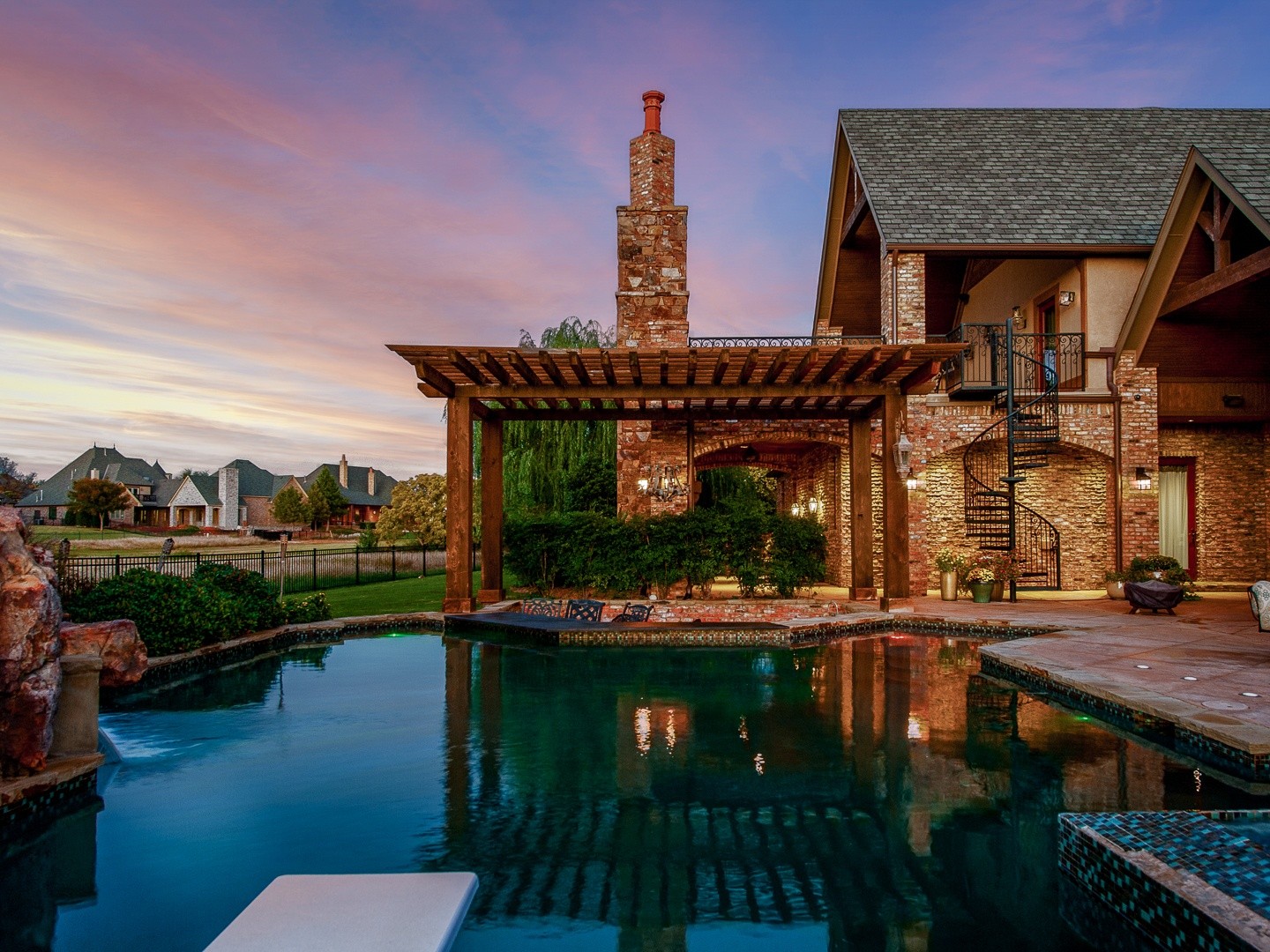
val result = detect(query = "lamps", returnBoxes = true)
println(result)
[808,490,828,515]
[742,443,760,463]
[1011,305,1027,330]
[1135,467,1151,490]
[1222,394,1245,409]
[792,497,807,518]
[636,463,691,503]
[892,410,914,481]
[1059,291,1075,306]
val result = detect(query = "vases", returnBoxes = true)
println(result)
[940,572,959,601]
[990,580,1004,602]
[968,578,994,603]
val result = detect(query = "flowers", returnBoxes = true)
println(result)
[965,568,995,583]
[933,547,966,573]
[956,548,1026,596]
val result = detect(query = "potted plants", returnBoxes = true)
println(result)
[1103,555,1204,616]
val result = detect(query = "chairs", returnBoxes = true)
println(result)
[620,602,654,622]
[521,598,563,618]
[565,598,606,622]
